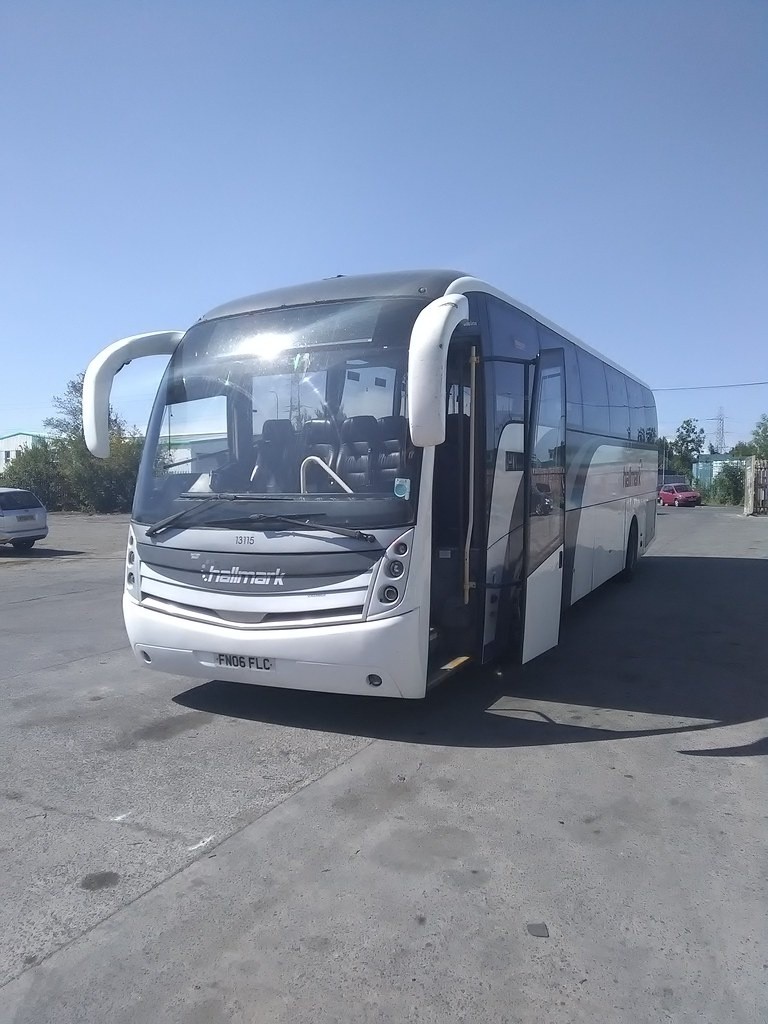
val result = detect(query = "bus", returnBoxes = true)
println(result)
[81,271,659,699]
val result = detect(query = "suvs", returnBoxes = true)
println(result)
[0,488,49,550]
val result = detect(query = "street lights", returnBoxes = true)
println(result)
[269,390,279,419]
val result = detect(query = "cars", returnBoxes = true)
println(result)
[660,483,702,507]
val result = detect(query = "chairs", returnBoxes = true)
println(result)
[248,412,471,493]
